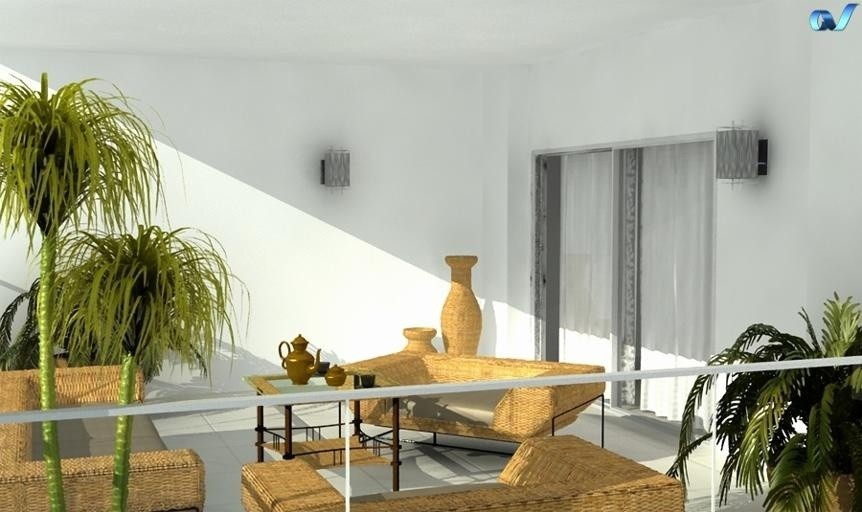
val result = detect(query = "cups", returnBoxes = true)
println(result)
[317,362,330,376]
[361,375,375,388]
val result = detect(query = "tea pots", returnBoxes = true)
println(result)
[279,334,321,385]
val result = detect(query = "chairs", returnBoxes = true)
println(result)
[241,435,686,512]
[0,365,205,512]
[336,351,605,455]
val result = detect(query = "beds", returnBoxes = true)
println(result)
[243,370,402,492]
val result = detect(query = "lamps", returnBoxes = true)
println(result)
[319,144,350,194]
[715,122,768,190]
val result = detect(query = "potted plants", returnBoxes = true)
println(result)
[665,290,862,512]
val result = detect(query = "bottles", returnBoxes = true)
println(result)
[325,364,346,386]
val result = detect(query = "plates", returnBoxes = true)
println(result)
[355,386,381,390]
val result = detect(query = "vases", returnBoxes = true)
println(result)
[440,256,482,355]
[402,327,438,353]
